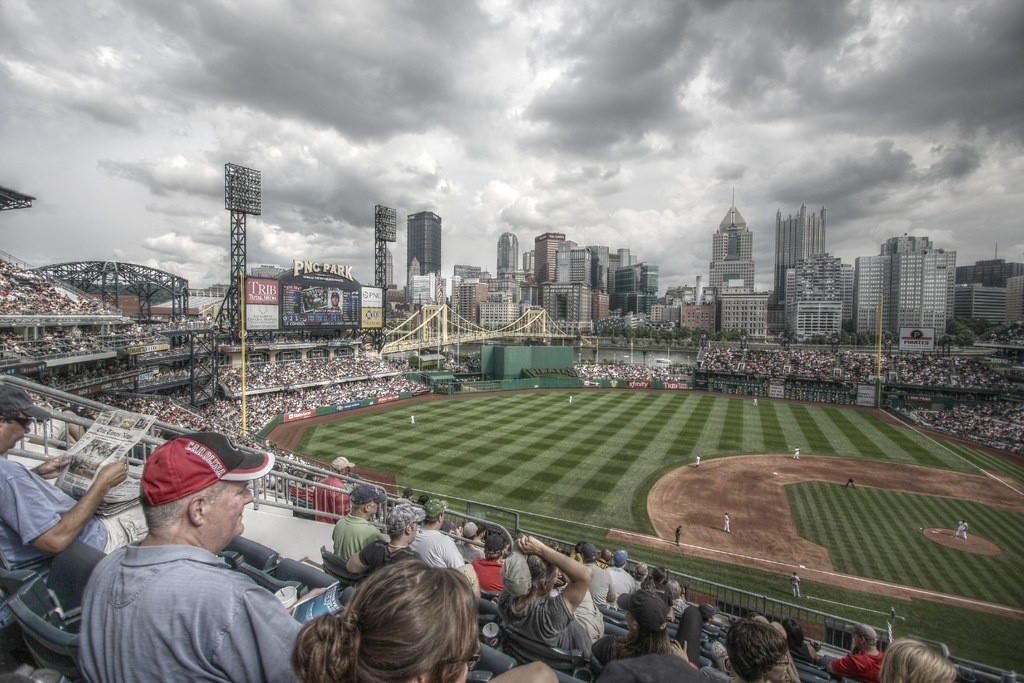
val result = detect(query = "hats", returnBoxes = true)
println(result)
[580,542,599,561]
[349,483,387,505]
[422,498,449,522]
[330,456,356,471]
[463,521,479,538]
[441,519,455,532]
[138,431,276,507]
[385,503,426,534]
[484,532,508,554]
[845,623,878,644]
[613,549,628,565]
[698,602,720,621]
[616,588,668,633]
[499,539,532,598]
[0,383,51,421]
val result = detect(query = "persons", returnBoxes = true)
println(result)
[789,571,801,598]
[696,455,700,468]
[0,257,481,439]
[569,395,572,403]
[411,414,414,424]
[955,520,968,538]
[0,384,957,683]
[753,397,757,405]
[792,446,800,459]
[722,513,731,533]
[675,525,682,546]
[845,478,855,488]
[574,324,1024,452]
[330,292,340,310]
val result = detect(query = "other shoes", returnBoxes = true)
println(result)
[710,640,731,675]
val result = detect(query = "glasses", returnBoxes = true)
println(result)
[775,654,790,665]
[435,636,482,671]
[2,416,35,428]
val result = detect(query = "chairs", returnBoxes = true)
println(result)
[0,546,850,683]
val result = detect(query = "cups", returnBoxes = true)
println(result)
[275,586,297,609]
[483,622,500,648]
